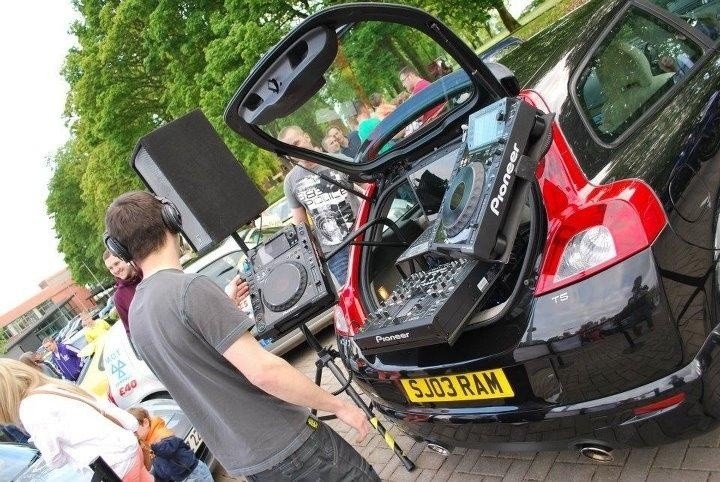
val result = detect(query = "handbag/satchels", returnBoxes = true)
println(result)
[134,431,154,472]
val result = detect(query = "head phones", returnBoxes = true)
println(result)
[102,191,182,263]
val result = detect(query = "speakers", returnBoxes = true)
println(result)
[129,109,270,257]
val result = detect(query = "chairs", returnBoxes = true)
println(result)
[590,41,676,132]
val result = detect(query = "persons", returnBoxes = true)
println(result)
[104,191,382,482]
[0,337,215,482]
[82,312,110,343]
[278,66,444,284]
[102,252,144,362]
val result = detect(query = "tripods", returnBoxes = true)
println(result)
[232,231,416,473]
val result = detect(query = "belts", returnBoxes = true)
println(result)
[180,457,198,479]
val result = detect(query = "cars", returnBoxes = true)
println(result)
[0,195,330,482]
[224,0,719,463]
